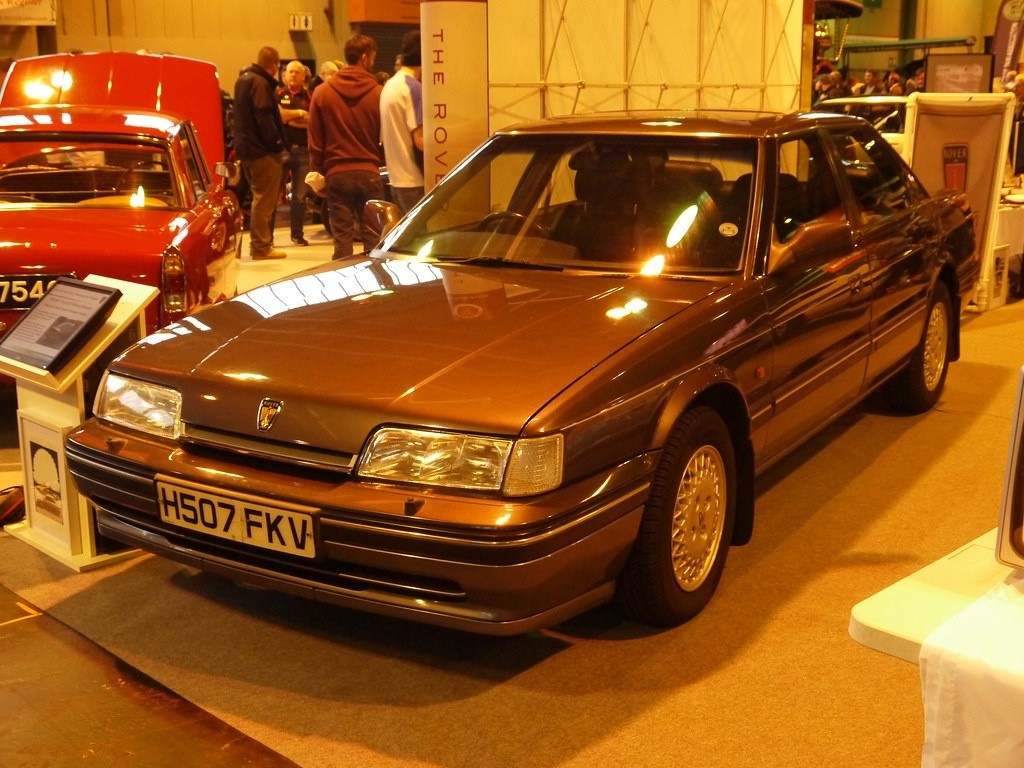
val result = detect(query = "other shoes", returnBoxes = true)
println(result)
[253,246,287,259]
[292,237,308,246]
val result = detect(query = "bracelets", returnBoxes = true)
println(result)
[298,109,302,117]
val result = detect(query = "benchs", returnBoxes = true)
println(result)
[658,160,835,242]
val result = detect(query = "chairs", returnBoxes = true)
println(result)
[550,152,656,263]
[705,174,805,271]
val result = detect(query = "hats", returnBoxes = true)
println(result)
[400,30,421,66]
[1005,74,1024,90]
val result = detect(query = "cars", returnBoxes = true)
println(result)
[62,109,981,643]
[0,51,246,399]
[812,93,911,168]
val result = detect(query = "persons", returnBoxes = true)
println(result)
[269,60,313,246]
[812,69,1024,174]
[233,31,424,260]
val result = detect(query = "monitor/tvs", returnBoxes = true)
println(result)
[0,277,123,374]
